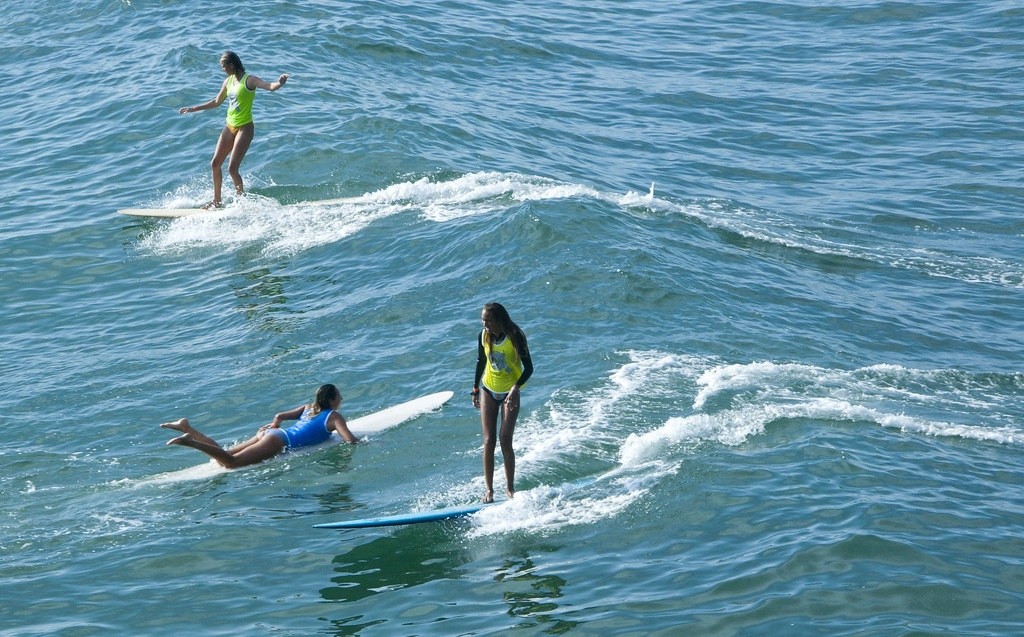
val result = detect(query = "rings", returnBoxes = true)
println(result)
[507,400,512,403]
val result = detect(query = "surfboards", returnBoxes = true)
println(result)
[132,390,456,488]
[118,194,381,219]
[312,472,608,532]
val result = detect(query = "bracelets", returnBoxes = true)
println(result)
[470,389,480,396]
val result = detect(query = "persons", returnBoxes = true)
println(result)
[179,51,290,210]
[470,302,534,504]
[160,383,359,468]
